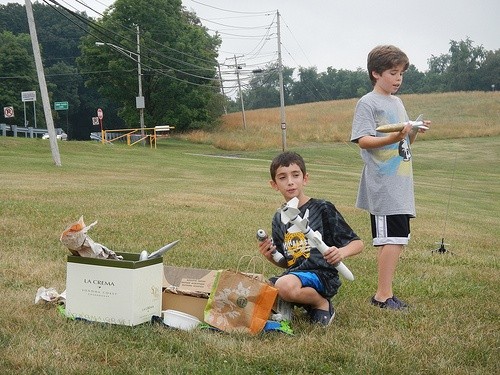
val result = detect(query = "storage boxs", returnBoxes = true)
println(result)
[65,251,264,327]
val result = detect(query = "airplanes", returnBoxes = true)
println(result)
[375,113,430,133]
[139,239,180,261]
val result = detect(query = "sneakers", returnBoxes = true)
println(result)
[310,299,335,327]
[370,294,409,311]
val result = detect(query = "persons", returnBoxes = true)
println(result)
[259,151,364,328]
[350,44,430,314]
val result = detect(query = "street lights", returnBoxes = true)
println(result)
[94,26,146,147]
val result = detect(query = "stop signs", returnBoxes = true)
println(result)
[97,109,104,119]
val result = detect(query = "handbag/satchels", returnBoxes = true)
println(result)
[204,255,278,338]
